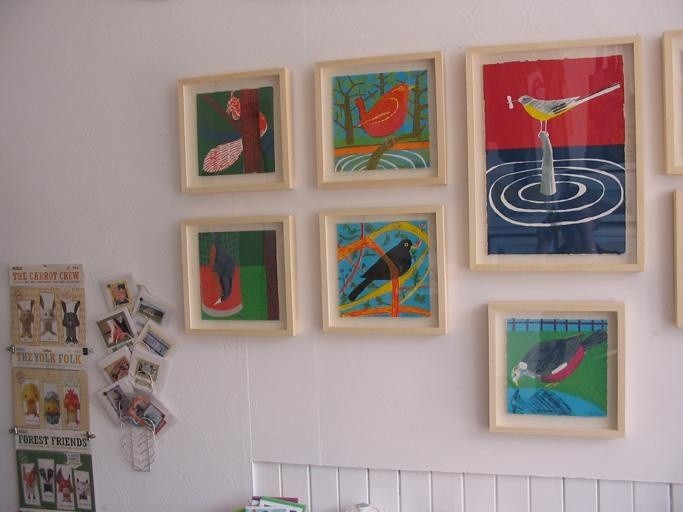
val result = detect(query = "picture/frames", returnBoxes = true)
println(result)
[672,191,682,327]
[176,67,296,194]
[465,35,646,272]
[179,215,299,337]
[318,204,449,336]
[313,50,447,191]
[662,29,683,176]
[486,301,625,438]
